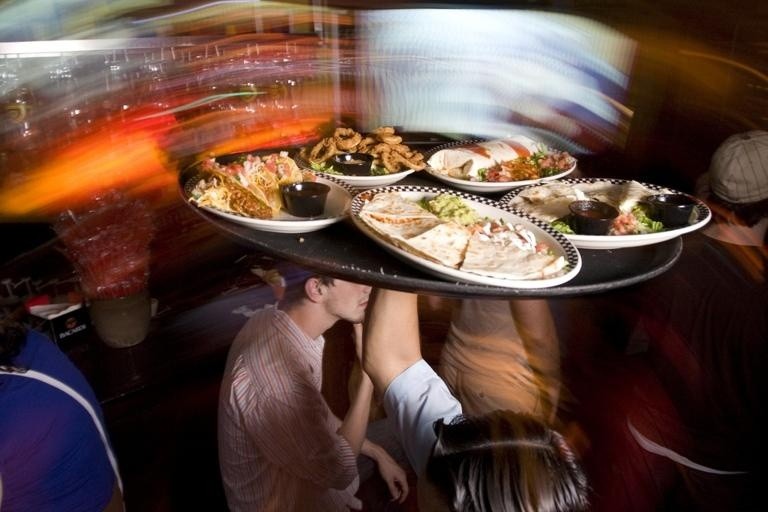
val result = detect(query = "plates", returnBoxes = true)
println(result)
[416,143,582,194]
[351,182,585,290]
[298,135,417,184]
[501,179,713,248]
[182,165,360,238]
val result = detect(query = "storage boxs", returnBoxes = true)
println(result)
[28,301,91,350]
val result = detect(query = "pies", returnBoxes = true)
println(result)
[358,192,567,281]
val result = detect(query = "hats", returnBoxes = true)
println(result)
[710,130,767,204]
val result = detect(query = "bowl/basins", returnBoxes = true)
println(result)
[640,194,695,227]
[333,153,374,176]
[568,201,620,235]
[284,182,330,217]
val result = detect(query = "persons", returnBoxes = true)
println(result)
[213,260,412,512]
[424,291,565,430]
[360,279,595,512]
[0,281,132,509]
[625,127,767,512]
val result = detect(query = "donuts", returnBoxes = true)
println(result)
[300,126,425,172]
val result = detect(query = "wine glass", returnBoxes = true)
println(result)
[1,35,308,134]
